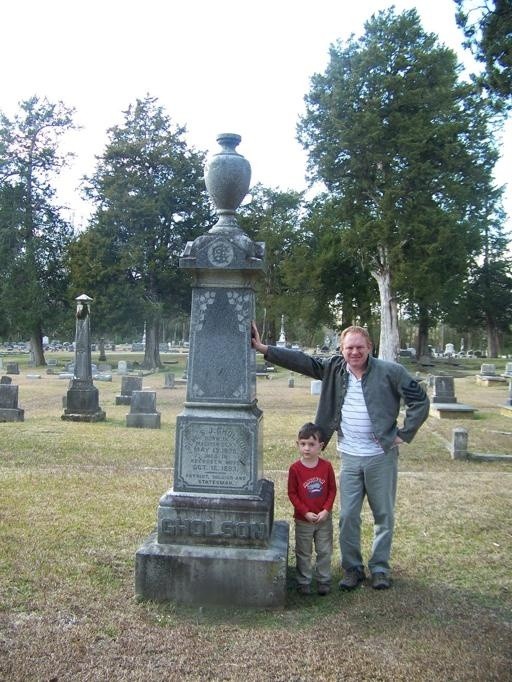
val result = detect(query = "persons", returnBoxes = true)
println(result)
[287,422,336,595]
[251,319,429,588]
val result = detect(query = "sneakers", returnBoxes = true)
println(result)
[372,571,390,588]
[318,581,331,595]
[297,584,311,594]
[338,568,365,588]
